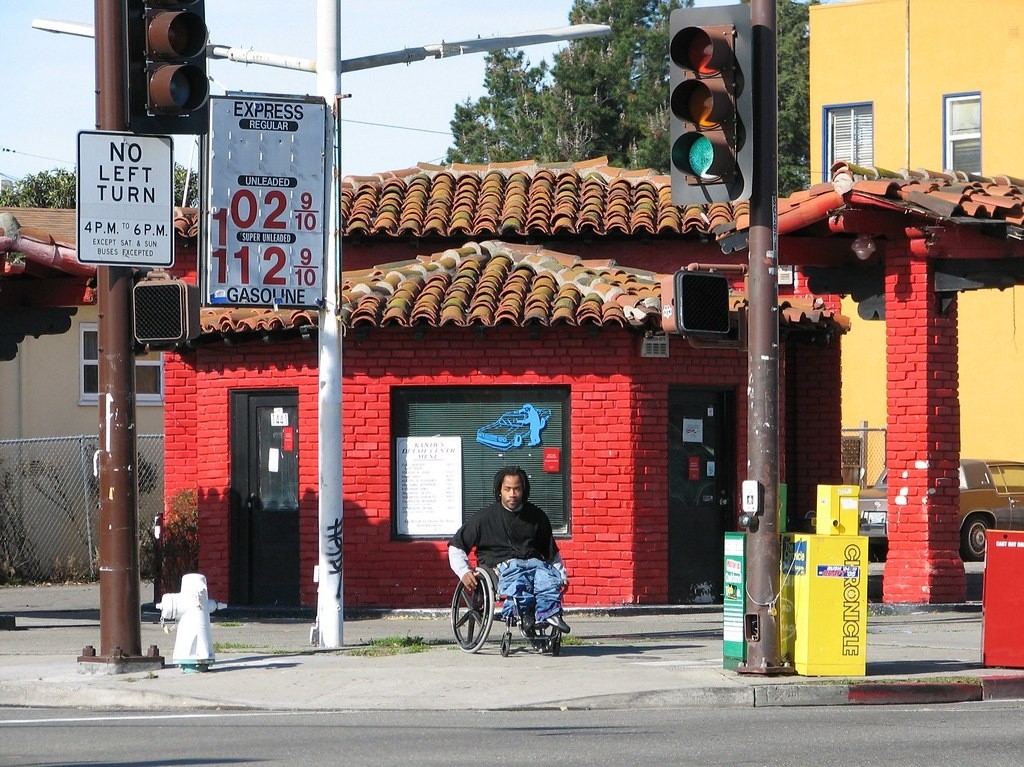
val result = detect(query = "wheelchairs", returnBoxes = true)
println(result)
[450,564,570,657]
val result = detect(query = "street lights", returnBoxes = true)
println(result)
[31,0,612,648]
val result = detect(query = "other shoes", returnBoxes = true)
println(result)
[545,613,570,633]
[520,614,535,631]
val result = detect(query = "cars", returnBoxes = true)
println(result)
[858,458,1024,562]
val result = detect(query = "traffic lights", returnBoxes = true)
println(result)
[660,269,731,337]
[122,0,210,135]
[669,4,754,206]
[131,271,202,345]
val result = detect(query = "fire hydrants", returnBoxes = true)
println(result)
[156,573,227,672]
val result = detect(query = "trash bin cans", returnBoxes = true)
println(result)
[723,483,787,672]
[778,484,869,677]
[981,529,1024,669]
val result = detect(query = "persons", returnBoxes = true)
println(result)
[447,467,570,638]
